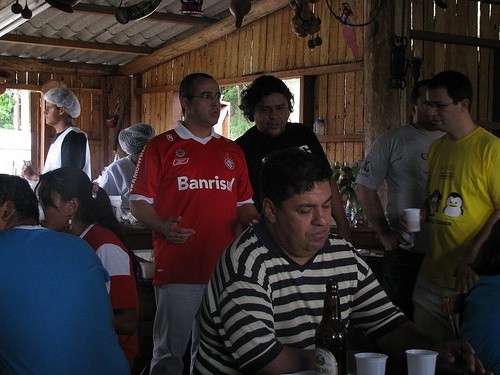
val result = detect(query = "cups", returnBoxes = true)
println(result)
[354,352,388,375]
[403,208,421,232]
[406,349,438,375]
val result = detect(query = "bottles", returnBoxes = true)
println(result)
[356,208,364,227]
[312,119,324,134]
[314,280,349,375]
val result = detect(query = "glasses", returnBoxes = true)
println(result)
[183,92,221,101]
[423,101,453,110]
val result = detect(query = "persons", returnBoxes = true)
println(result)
[352,78,447,322]
[461,217,500,375]
[128,72,259,375]
[37,166,141,360]
[0,174,133,375]
[235,75,352,245]
[399,69,500,343]
[94,123,156,196]
[190,147,475,375]
[20,87,94,180]
